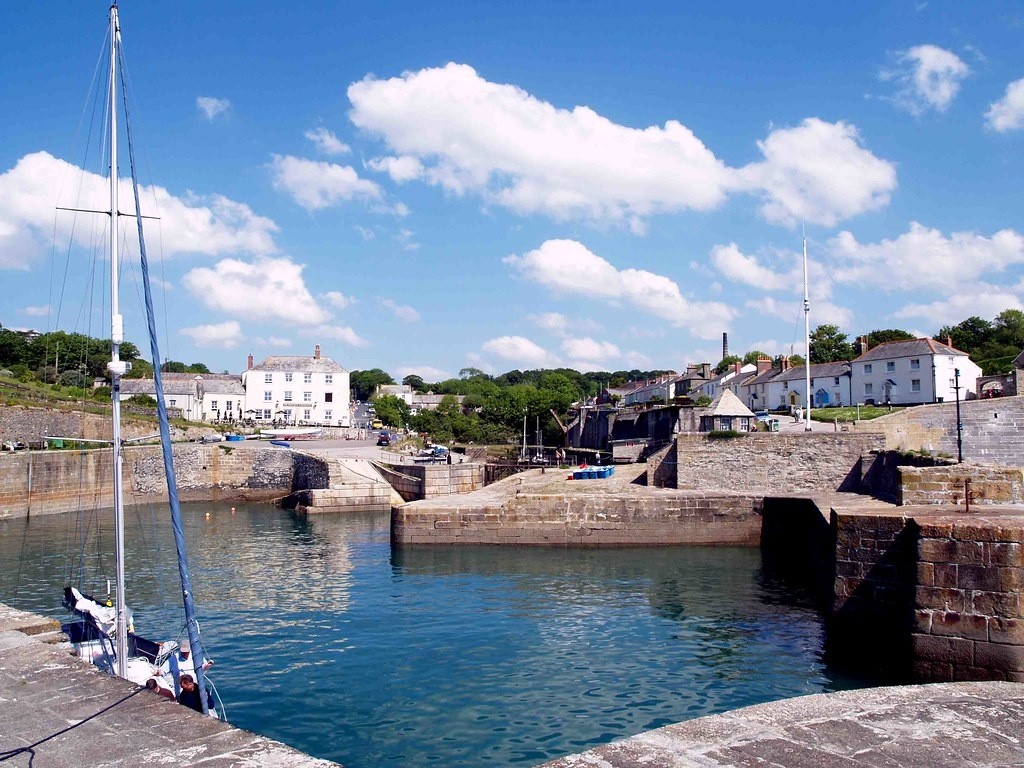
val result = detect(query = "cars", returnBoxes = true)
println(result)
[752,410,770,423]
[195,433,290,448]
[980,389,1004,398]
[363,415,450,462]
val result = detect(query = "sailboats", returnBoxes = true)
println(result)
[532,416,548,464]
[5,1,229,725]
[517,415,531,463]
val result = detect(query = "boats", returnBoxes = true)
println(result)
[258,425,322,440]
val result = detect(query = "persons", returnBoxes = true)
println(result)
[560,448,566,465]
[554,450,561,468]
[143,678,177,701]
[176,675,217,715]
